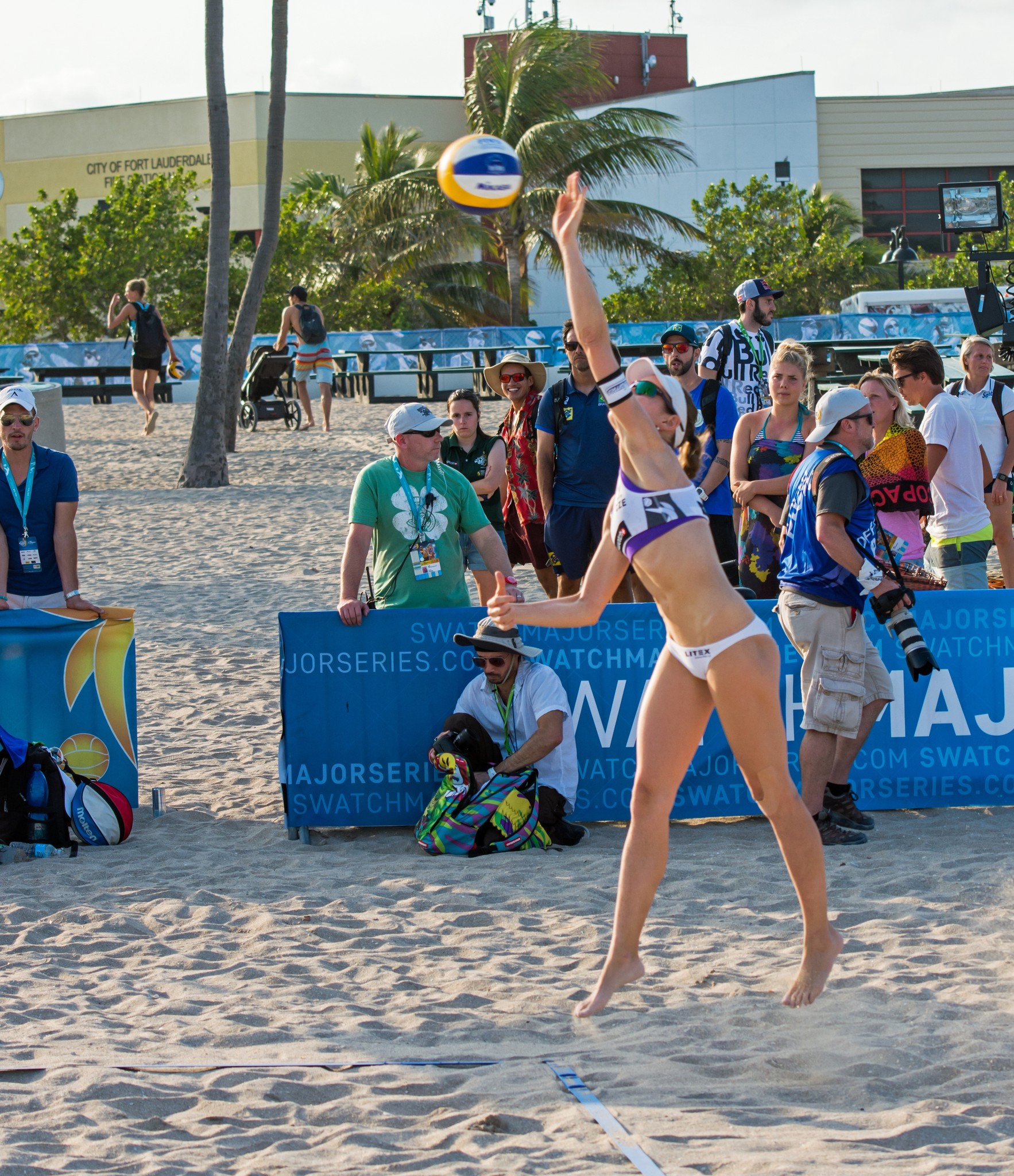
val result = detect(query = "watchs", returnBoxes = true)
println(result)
[996,473,1009,482]
[504,576,517,585]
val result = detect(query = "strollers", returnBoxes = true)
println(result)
[237,342,302,433]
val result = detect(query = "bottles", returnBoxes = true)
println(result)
[27,764,49,838]
[9,842,62,858]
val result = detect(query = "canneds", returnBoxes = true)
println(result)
[152,787,166,817]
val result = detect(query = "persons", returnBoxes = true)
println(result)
[272,286,333,433]
[336,401,525,628]
[486,168,842,1018]
[537,314,1014,603]
[698,278,786,421]
[0,385,105,615]
[770,386,915,845]
[427,616,580,845]
[484,352,558,603]
[438,388,508,607]
[107,278,181,437]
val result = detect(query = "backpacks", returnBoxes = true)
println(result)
[0,743,70,848]
[290,304,326,344]
[416,751,538,856]
[126,301,168,359]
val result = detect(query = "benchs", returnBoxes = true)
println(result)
[816,374,864,383]
[902,399,926,429]
[817,383,849,397]
[62,382,182,405]
[309,362,554,404]
[558,364,698,376]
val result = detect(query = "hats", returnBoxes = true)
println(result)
[0,385,37,415]
[468,329,488,338]
[483,353,547,398]
[660,323,698,348]
[287,285,307,297]
[360,334,375,344]
[804,387,870,442]
[802,319,821,327]
[733,279,784,304]
[384,402,454,444]
[24,345,39,354]
[939,317,953,325]
[454,616,542,657]
[625,357,688,448]
[84,350,101,361]
[884,317,898,328]
[610,333,618,337]
[694,322,709,332]
[420,337,436,348]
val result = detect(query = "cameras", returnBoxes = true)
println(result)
[870,586,941,683]
[433,727,480,773]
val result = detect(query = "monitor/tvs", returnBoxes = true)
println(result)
[937,181,1004,233]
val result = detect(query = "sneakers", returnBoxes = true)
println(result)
[812,811,867,846]
[822,786,874,830]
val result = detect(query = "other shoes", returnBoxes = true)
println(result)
[561,818,589,840]
[143,412,158,436]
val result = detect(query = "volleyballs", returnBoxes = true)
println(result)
[435,132,525,217]
[167,362,184,379]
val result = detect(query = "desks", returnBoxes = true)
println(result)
[331,354,359,398]
[24,365,169,405]
[558,342,703,375]
[338,345,551,404]
[774,334,1014,398]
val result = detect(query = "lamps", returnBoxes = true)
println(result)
[964,260,1007,337]
[937,180,1010,253]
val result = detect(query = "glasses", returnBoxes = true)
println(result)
[661,342,698,353]
[810,325,818,329]
[564,341,583,351]
[628,380,685,432]
[0,414,36,426]
[848,412,873,425]
[894,373,912,387]
[87,355,91,359]
[553,337,560,341]
[888,323,899,328]
[26,353,39,358]
[500,373,527,383]
[362,343,375,347]
[402,427,441,438]
[476,335,486,340]
[421,342,427,346]
[696,330,706,335]
[471,652,510,667]
[610,337,616,340]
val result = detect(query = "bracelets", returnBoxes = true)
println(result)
[63,589,81,601]
[0,595,8,601]
[595,366,634,410]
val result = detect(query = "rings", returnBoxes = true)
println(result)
[999,496,1003,497]
[517,595,522,598]
